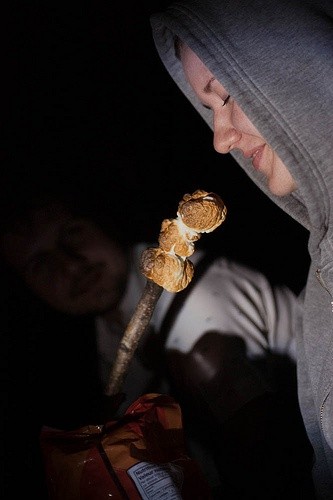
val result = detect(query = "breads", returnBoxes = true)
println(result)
[140,189,228,292]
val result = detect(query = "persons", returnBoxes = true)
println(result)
[0,180,298,500]
[149,0,333,500]
